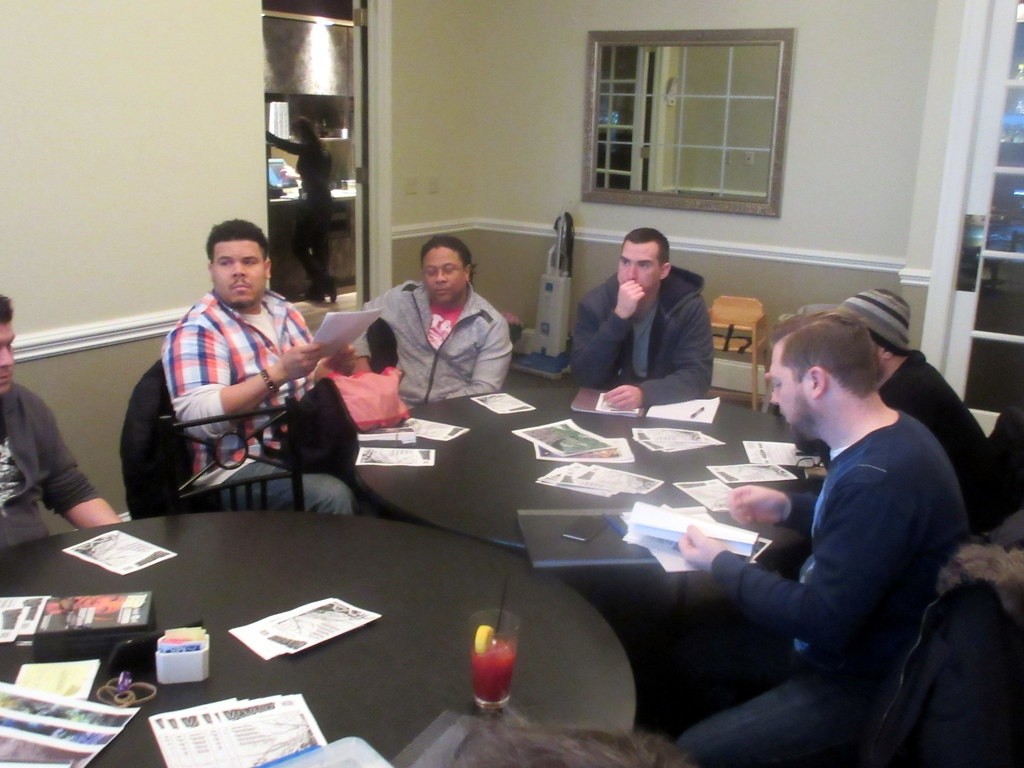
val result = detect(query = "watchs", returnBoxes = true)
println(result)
[261,369,279,392]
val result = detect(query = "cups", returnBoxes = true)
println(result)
[341,180,356,190]
[269,102,289,139]
[468,609,520,709]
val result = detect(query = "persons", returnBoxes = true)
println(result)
[676,306,1024,768]
[161,220,356,517]
[0,295,123,554]
[573,227,714,409]
[351,235,513,411]
[267,114,338,304]
[441,719,698,768]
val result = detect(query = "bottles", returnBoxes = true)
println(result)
[341,117,348,139]
[313,117,328,137]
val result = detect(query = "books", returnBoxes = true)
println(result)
[572,383,645,418]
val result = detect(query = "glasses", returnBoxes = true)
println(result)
[797,458,827,470]
[422,264,464,279]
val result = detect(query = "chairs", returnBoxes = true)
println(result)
[121,360,357,516]
[161,395,306,515]
[860,508,1024,768]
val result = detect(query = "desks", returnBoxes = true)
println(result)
[354,387,821,606]
[0,508,636,768]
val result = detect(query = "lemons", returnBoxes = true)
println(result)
[475,625,494,654]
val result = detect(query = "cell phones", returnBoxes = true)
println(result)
[107,621,203,675]
[562,516,609,543]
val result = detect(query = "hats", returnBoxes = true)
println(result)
[841,287,911,358]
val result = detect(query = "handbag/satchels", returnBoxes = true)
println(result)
[283,376,359,479]
[324,367,411,428]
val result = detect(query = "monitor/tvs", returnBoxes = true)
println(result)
[268,159,299,189]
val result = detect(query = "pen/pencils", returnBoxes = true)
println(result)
[365,427,401,435]
[690,406,704,418]
[602,513,629,542]
